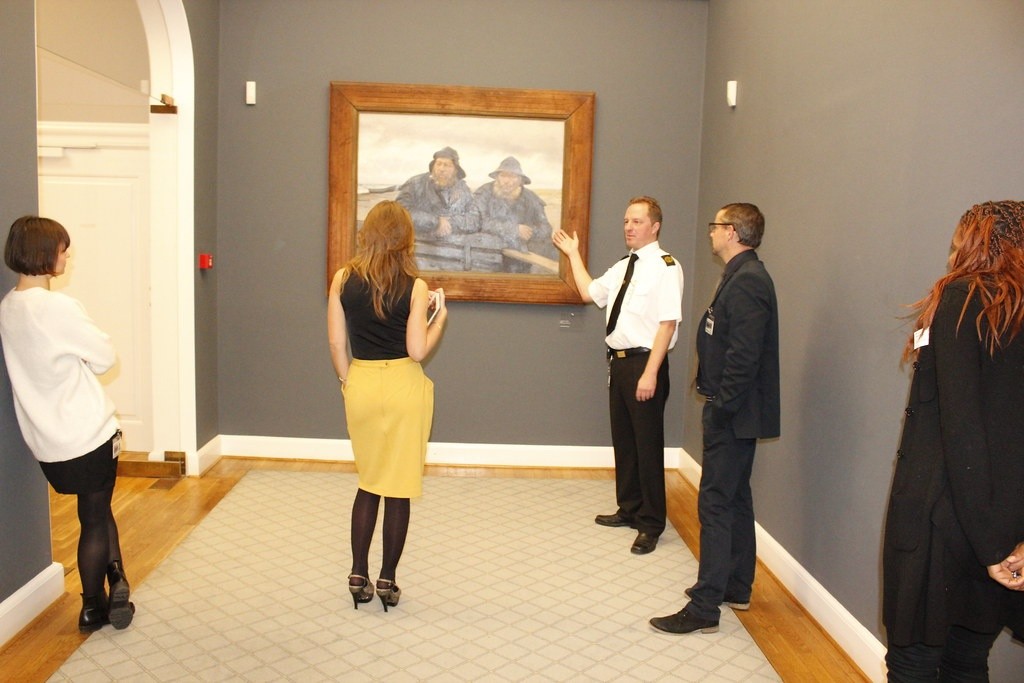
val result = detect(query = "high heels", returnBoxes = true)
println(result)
[350,574,375,608]
[376,578,401,613]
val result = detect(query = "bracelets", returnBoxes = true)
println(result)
[432,322,442,330]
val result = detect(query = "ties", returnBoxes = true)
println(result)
[606,254,639,337]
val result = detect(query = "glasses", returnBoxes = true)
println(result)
[708,222,736,231]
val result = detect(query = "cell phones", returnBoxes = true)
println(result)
[427,293,440,325]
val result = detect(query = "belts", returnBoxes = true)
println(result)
[611,347,651,360]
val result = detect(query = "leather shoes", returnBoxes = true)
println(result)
[686,586,751,610]
[108,560,133,631]
[648,602,720,635]
[595,514,634,526]
[631,531,660,555]
[79,588,134,632]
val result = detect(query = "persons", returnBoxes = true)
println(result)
[553,195,684,555]
[882,201,1024,683]
[328,200,447,613]
[648,202,781,634]
[0,215,135,632]
